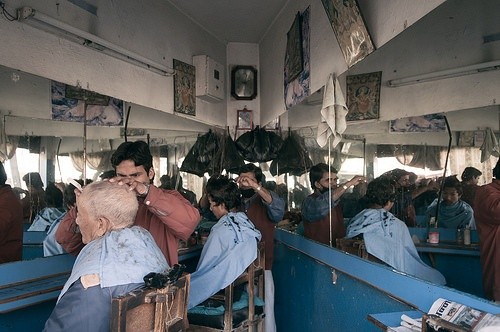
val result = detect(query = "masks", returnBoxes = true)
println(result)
[317,182,330,194]
[240,187,256,197]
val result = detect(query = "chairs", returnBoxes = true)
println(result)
[336,237,368,260]
[185,252,268,331]
[110,274,190,332]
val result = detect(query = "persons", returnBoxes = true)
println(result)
[41,181,171,332]
[177,175,262,308]
[262,172,312,211]
[301,158,500,304]
[0,161,117,264]
[199,163,285,332]
[158,174,197,206]
[55,141,200,268]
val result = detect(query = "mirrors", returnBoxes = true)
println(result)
[0,63,227,265]
[270,103,499,231]
[3,114,215,227]
[266,0,500,303]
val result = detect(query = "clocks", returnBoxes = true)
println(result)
[228,64,258,101]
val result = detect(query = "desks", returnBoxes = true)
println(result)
[0,275,82,315]
[270,229,499,332]
[22,232,50,247]
[414,241,481,256]
[1,251,79,288]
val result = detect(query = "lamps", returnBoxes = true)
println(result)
[384,59,500,87]
[18,7,176,76]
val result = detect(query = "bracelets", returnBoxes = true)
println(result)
[139,182,149,197]
[254,184,261,192]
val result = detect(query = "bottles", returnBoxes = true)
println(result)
[456,224,471,245]
